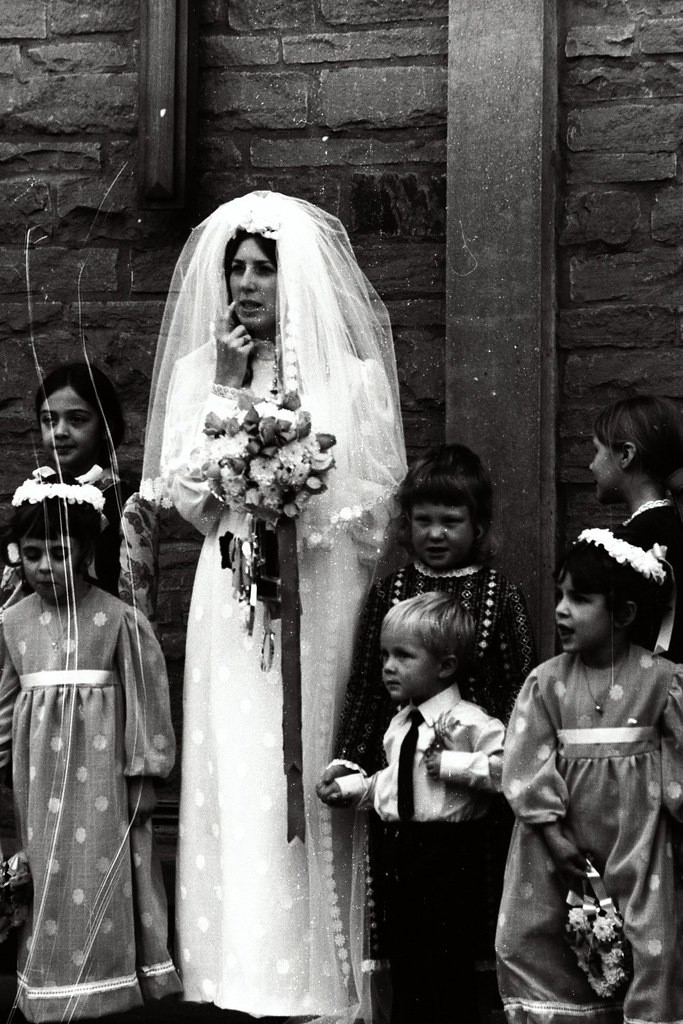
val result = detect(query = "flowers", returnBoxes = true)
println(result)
[562,899,628,998]
[181,389,338,533]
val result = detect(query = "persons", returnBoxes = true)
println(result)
[138,188,412,1024]
[2,461,184,1018]
[0,358,158,897]
[588,395,683,658]
[320,590,516,1023]
[313,437,533,1023]
[490,522,683,1024]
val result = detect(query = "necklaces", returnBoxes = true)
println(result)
[39,595,85,652]
[578,652,628,712]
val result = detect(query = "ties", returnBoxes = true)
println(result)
[396,709,426,820]
